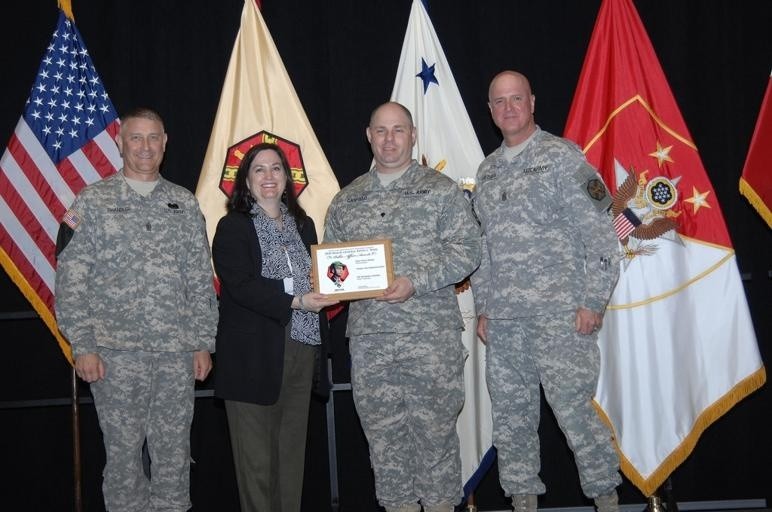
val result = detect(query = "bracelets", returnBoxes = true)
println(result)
[298,296,309,316]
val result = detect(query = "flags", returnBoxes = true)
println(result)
[1,1,136,378]
[560,1,768,502]
[368,2,499,502]
[734,74,771,231]
[189,2,358,329]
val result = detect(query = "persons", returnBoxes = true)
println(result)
[208,142,350,510]
[54,107,222,511]
[329,263,344,281]
[464,69,625,512]
[323,98,486,512]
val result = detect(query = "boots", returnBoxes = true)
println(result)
[384,503,455,512]
[509,493,537,512]
[594,493,619,512]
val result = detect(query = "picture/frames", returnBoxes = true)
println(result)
[310,239,396,301]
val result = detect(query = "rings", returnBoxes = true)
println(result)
[594,326,598,332]
